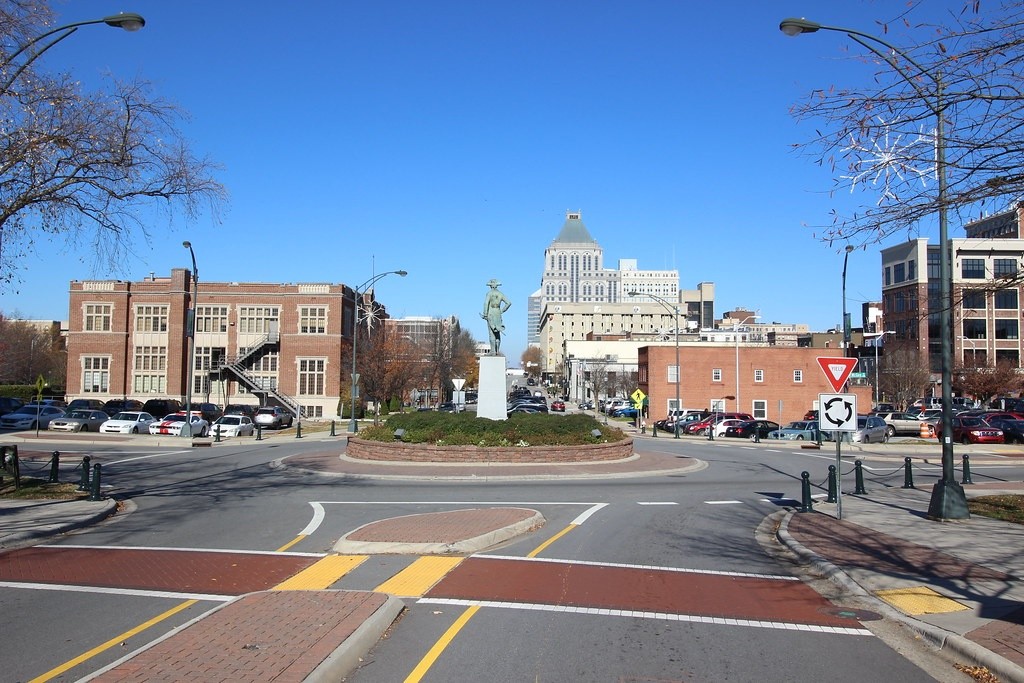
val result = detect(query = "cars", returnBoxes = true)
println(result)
[507,372,550,419]
[767,419,834,442]
[655,410,759,438]
[725,420,784,440]
[208,414,255,438]
[867,395,1024,445]
[25,398,254,426]
[578,397,644,418]
[48,408,110,432]
[99,410,156,435]
[149,410,209,438]
[551,400,566,412]
[0,396,30,417]
[416,391,478,413]
[0,404,66,431]
[839,414,889,444]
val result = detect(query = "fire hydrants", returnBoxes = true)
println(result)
[640,421,646,434]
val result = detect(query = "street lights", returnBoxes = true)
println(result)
[347,269,408,432]
[628,291,681,438]
[603,376,608,426]
[777,16,970,526]
[179,239,199,437]
[562,378,565,400]
[735,315,763,413]
[540,355,548,388]
[875,331,898,411]
[957,336,978,406]
[840,244,855,442]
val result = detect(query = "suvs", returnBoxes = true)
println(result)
[253,404,294,430]
[803,409,819,420]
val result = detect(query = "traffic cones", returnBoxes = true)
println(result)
[930,427,936,439]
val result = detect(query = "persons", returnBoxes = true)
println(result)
[702,408,709,420]
[482,278,512,356]
